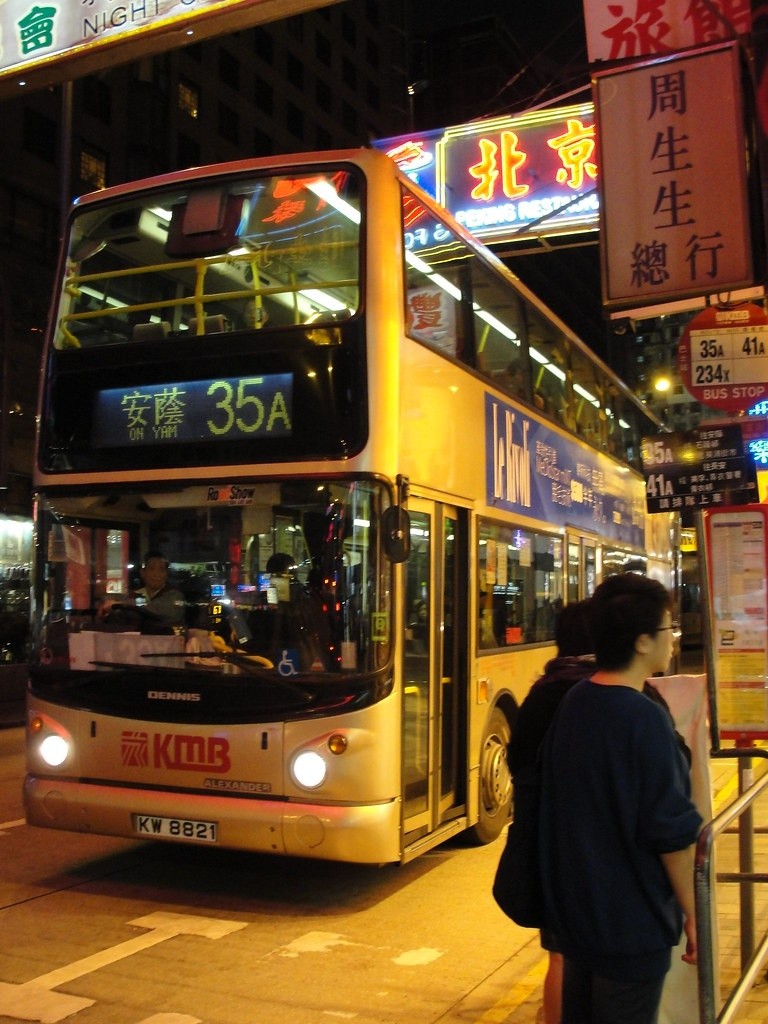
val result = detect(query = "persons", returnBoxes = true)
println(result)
[98,552,185,624]
[242,298,269,329]
[405,599,429,639]
[246,553,309,640]
[503,359,546,409]
[507,572,702,1024]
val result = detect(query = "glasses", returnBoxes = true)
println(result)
[652,621,685,637]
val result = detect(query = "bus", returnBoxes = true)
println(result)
[22,149,681,865]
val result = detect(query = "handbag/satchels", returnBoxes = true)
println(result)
[492,822,553,929]
